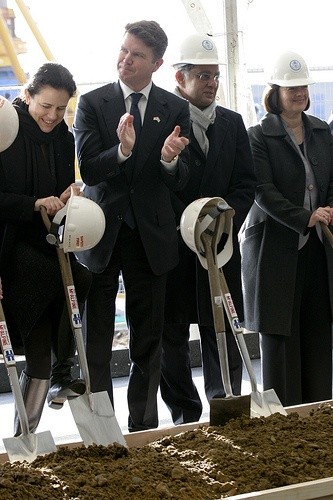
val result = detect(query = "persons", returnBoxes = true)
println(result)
[159,33,257,426]
[73,19,192,432]
[238,51,333,407]
[0,62,86,437]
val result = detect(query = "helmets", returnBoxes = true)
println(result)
[180,196,234,271]
[47,186,106,253]
[171,36,227,67]
[266,53,320,88]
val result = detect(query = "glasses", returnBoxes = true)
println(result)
[185,70,222,81]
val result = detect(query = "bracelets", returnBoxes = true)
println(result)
[162,155,177,163]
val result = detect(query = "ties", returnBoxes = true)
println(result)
[130,93,143,153]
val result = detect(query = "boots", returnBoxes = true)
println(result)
[48,327,86,409]
[8,370,51,437]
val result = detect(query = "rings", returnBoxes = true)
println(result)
[323,216,325,217]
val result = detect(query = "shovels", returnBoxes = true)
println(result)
[202,214,251,427]
[320,221,333,248]
[218,267,288,417]
[0,301,57,464]
[40,206,129,450]
